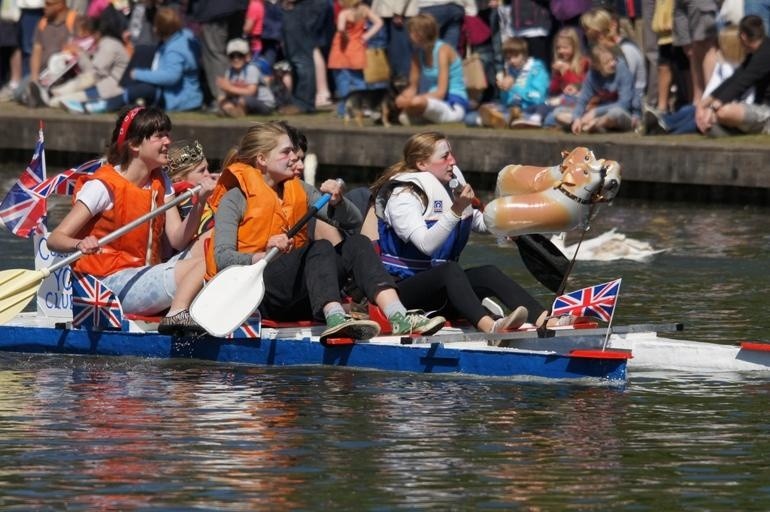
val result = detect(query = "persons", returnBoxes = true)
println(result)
[416,1,464,50]
[371,0,421,89]
[473,1,504,103]
[694,14,769,138]
[497,0,555,78]
[510,29,592,129]
[582,9,631,71]
[546,77,580,121]
[646,24,755,135]
[608,0,641,24]
[620,17,636,41]
[552,0,591,29]
[718,0,745,26]
[376,130,572,347]
[555,47,634,133]
[45,105,214,334]
[329,0,384,127]
[670,0,719,106]
[651,0,678,112]
[381,15,467,126]
[611,14,647,127]
[640,0,659,107]
[162,138,222,201]
[213,119,447,346]
[280,119,381,256]
[479,39,549,128]
[745,1,770,36]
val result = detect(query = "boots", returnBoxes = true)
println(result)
[390,308,446,336]
[320,313,381,348]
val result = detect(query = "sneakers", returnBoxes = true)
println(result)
[59,99,81,112]
[647,109,670,136]
[488,306,528,347]
[30,78,51,109]
[510,119,542,130]
[556,316,591,326]
[157,311,203,334]
[480,104,505,128]
[0,82,14,104]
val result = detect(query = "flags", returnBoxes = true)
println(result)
[32,155,107,197]
[72,265,124,330]
[0,131,50,238]
[550,280,622,322]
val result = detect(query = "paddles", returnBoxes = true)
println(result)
[188,177,345,339]
[449,178,571,295]
[0,183,202,322]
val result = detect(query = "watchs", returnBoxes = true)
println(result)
[707,104,716,110]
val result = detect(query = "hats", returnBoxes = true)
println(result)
[226,38,249,57]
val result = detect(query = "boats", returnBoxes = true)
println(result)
[0,312,634,391]
[0,311,767,384]
